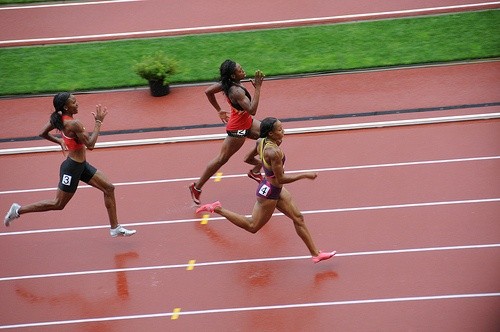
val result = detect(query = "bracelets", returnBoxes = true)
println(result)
[95,120,103,125]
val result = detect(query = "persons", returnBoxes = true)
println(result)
[195,116,337,264]
[188,60,265,205]
[4,92,137,238]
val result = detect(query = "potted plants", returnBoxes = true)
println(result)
[130,54,185,97]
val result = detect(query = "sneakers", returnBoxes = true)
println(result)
[196,201,222,214]
[189,183,202,204]
[110,226,136,237]
[4,203,21,227]
[312,251,337,263]
[248,170,263,183]
